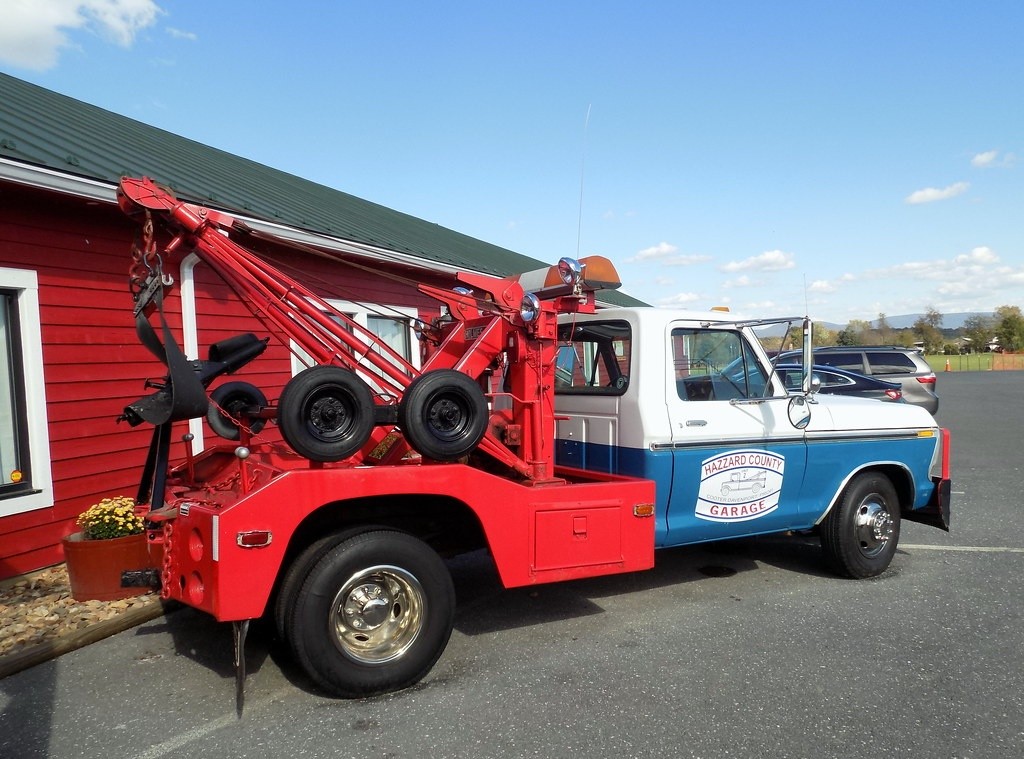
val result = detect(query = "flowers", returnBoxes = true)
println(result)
[75,495,145,539]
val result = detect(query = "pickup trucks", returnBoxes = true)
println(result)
[109,174,954,699]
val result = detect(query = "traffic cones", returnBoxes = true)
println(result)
[943,359,952,372]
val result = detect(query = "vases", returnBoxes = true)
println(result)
[63,528,156,601]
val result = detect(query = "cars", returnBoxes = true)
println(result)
[674,342,940,416]
